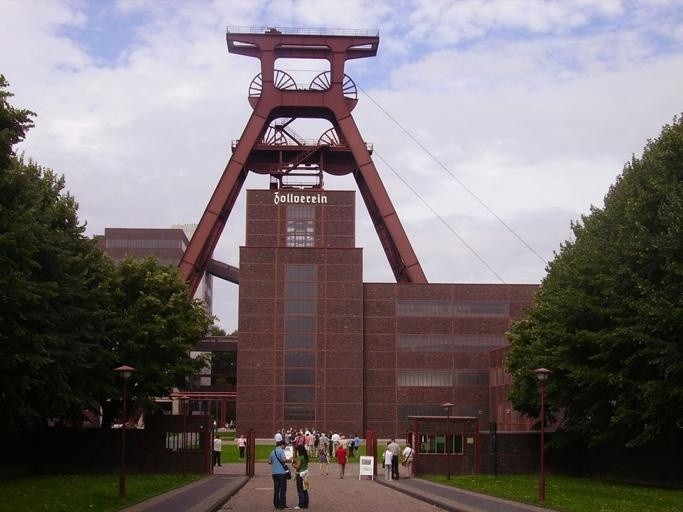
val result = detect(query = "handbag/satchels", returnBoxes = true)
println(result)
[284,464,291,480]
[302,479,310,491]
[402,457,407,466]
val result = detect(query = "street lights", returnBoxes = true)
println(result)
[177,394,192,480]
[534,367,553,503]
[112,365,137,500]
[440,402,456,480]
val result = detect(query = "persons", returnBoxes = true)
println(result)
[334,443,349,479]
[236,434,247,458]
[386,441,400,479]
[291,444,309,510]
[400,443,416,478]
[266,439,294,510]
[317,444,330,476]
[212,435,223,467]
[381,447,394,481]
[273,427,361,458]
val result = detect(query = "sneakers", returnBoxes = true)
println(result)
[278,504,302,509]
